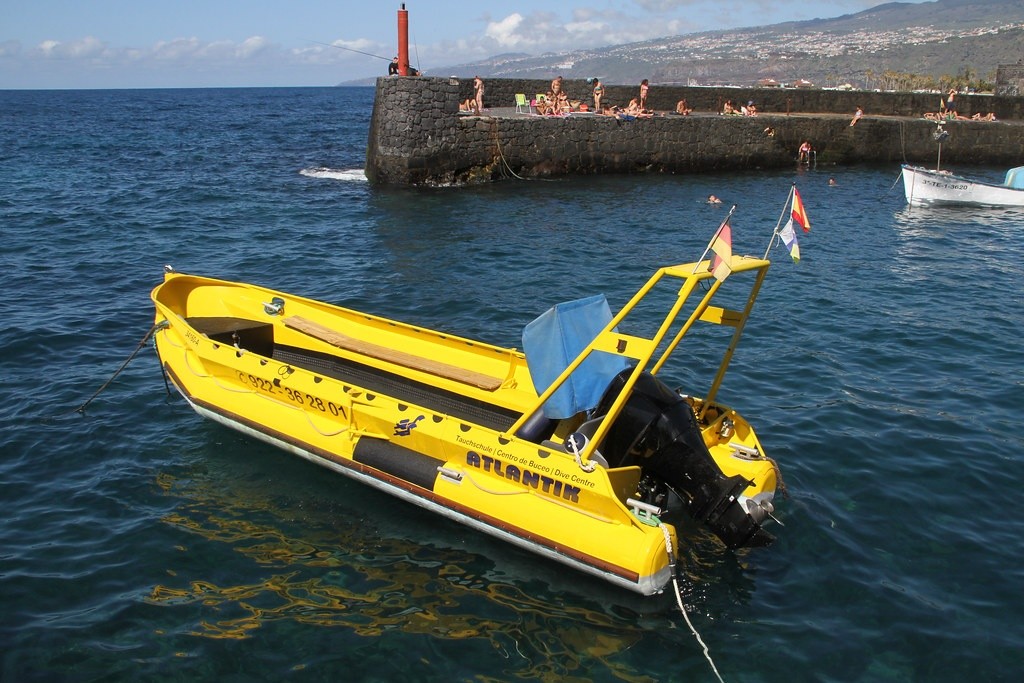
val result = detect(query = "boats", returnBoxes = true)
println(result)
[150,181,812,594]
[901,99,1024,208]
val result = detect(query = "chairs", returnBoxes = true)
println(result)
[515,93,550,114]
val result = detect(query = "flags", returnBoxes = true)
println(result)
[792,188,811,233]
[779,218,800,264]
[707,224,732,283]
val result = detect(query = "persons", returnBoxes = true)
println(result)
[850,106,864,127]
[676,98,693,115]
[589,78,668,116]
[924,89,996,121]
[799,140,811,160]
[389,57,398,75]
[456,76,484,112]
[829,178,835,185]
[532,76,572,118]
[708,195,721,203]
[724,100,756,116]
[764,126,774,138]
